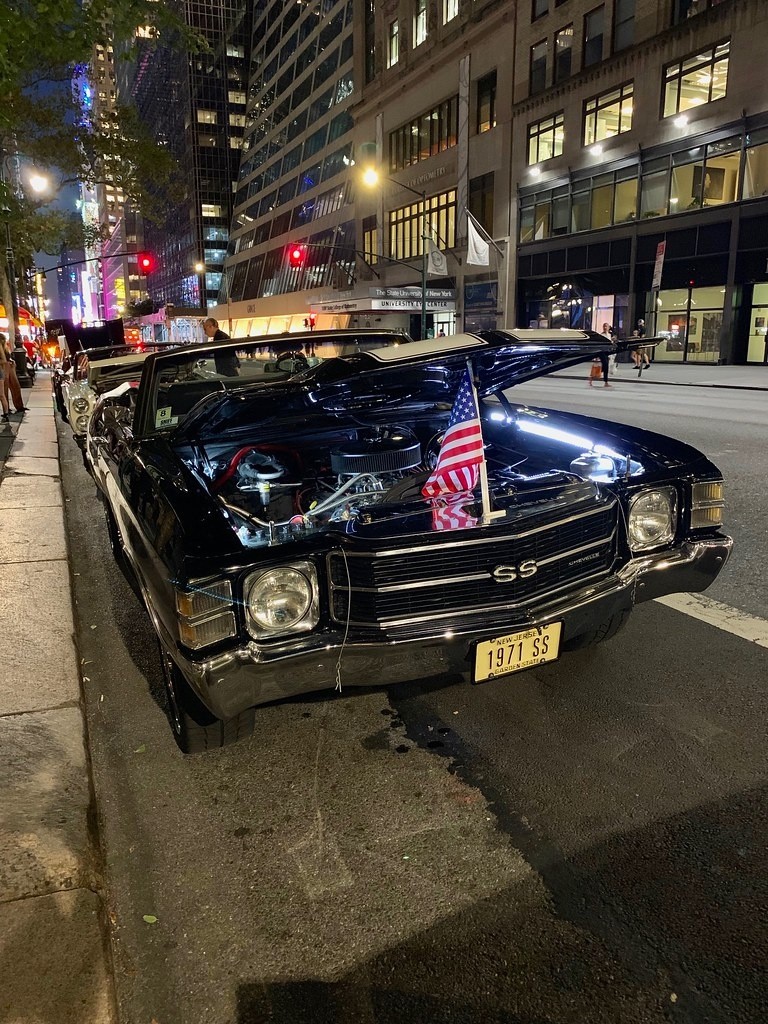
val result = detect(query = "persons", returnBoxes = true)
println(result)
[590,323,618,387]
[0,334,29,422]
[695,173,712,198]
[630,319,650,369]
[136,342,147,353]
[22,335,39,362]
[203,318,241,377]
[666,322,684,351]
[439,329,445,337]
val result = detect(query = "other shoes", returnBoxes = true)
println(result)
[633,366,641,369]
[589,381,593,386]
[633,365,636,368]
[604,384,612,387]
[1,413,9,418]
[16,407,30,412]
[643,364,650,369]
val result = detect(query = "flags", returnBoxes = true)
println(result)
[421,360,483,508]
[467,215,490,266]
[427,238,449,275]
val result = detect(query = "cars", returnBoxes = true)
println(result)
[51,326,733,754]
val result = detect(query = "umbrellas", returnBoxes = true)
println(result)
[0,304,45,327]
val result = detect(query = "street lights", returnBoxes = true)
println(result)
[194,263,232,337]
[0,148,49,388]
[361,168,428,341]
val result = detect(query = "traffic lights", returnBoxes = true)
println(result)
[139,254,152,275]
[310,313,315,327]
[290,248,305,268]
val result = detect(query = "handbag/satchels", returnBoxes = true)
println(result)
[590,359,602,379]
